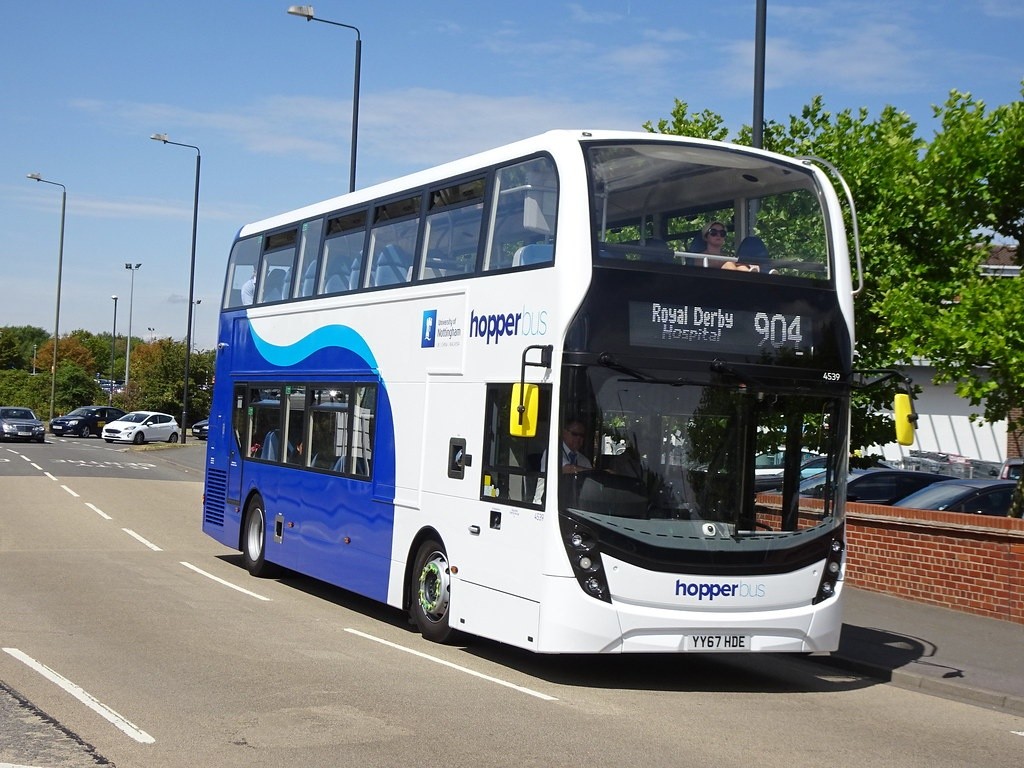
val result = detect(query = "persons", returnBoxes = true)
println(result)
[240,262,270,305]
[694,220,760,273]
[290,440,308,465]
[532,418,592,505]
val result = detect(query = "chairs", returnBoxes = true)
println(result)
[263,230,772,301]
[260,429,362,474]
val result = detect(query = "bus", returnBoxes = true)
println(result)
[204,126,918,659]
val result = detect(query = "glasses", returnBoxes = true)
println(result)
[564,427,586,438]
[708,229,727,237]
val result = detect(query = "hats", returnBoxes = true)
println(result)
[701,221,727,241]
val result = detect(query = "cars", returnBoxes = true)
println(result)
[0,406,45,443]
[191,418,209,440]
[101,411,180,445]
[892,477,1017,519]
[751,447,894,492]
[761,468,960,507]
[49,406,127,438]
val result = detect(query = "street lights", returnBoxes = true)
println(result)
[150,133,201,445]
[287,5,362,193]
[110,294,118,405]
[26,173,67,420]
[125,263,142,385]
[192,299,202,352]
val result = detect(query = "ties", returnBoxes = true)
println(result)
[568,451,578,465]
